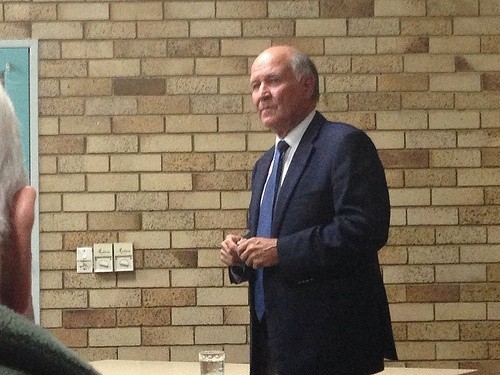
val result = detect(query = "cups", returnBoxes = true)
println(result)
[199,350,225,375]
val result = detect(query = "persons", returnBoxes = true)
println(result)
[221,46,400,375]
[0,82,103,375]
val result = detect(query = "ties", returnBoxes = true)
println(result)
[253,140,291,322]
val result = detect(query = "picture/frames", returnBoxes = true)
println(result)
[1,39,40,328]
